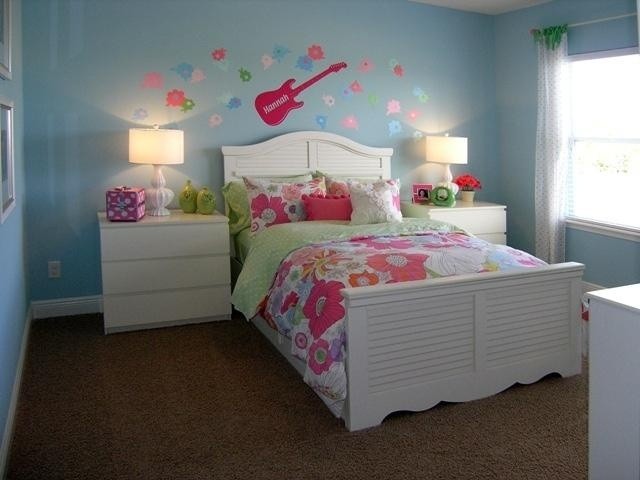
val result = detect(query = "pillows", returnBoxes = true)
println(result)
[349,178,402,225]
[316,170,352,196]
[302,192,351,220]
[221,172,317,236]
[242,175,326,237]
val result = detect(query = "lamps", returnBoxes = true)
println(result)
[128,124,185,218]
[426,133,468,195]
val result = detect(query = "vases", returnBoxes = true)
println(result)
[460,190,475,202]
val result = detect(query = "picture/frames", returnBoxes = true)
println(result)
[0,0,13,81]
[411,183,432,204]
[0,94,16,227]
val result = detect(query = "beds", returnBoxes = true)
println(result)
[220,130,586,433]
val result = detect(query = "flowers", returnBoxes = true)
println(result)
[454,174,482,191]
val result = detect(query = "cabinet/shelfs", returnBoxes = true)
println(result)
[96,209,233,335]
[401,199,507,246]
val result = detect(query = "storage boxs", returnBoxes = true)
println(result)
[105,185,146,222]
